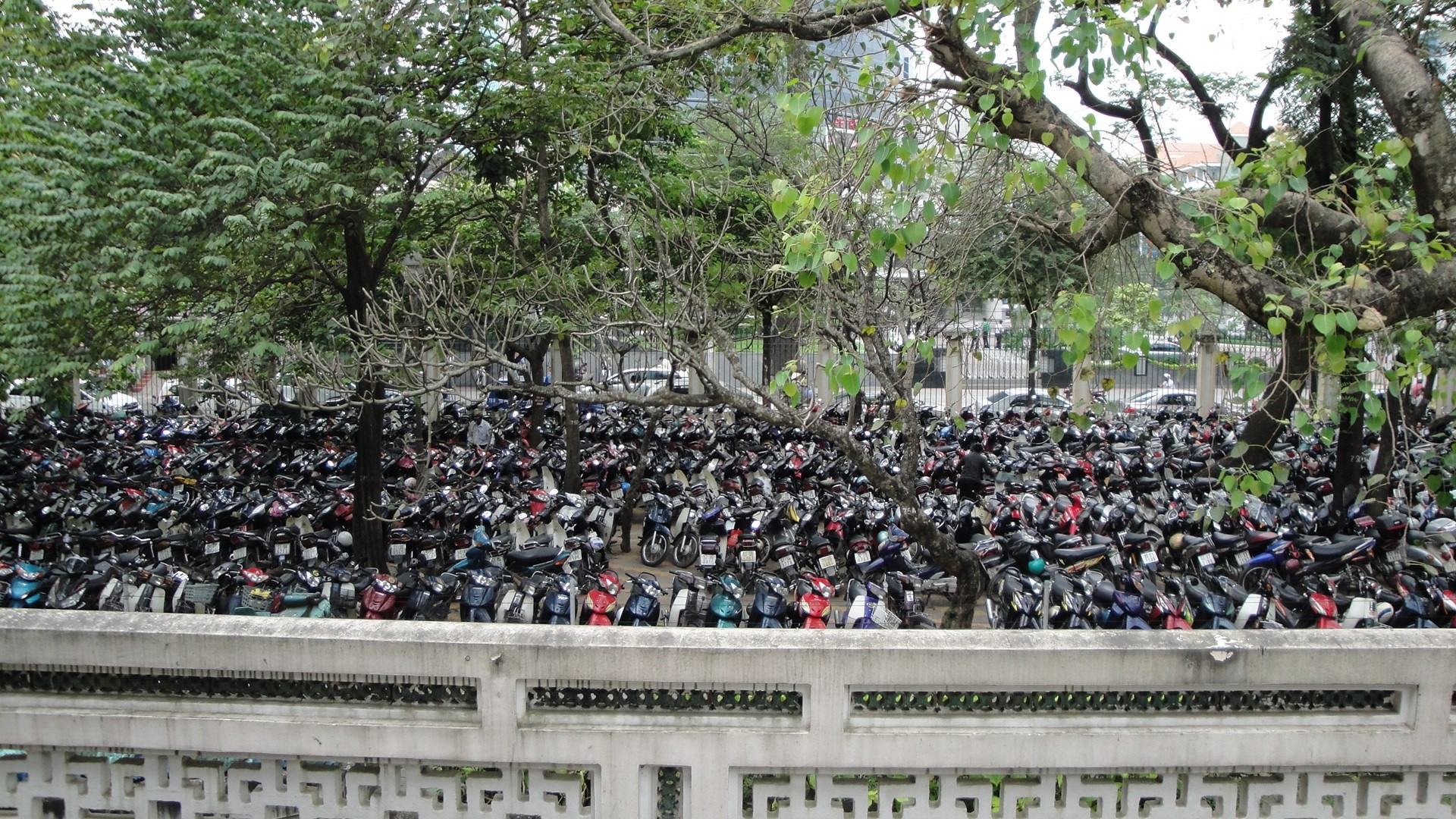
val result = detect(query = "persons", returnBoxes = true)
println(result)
[466,408,495,451]
[954,444,999,500]
[1366,436,1381,475]
[1412,378,1426,408]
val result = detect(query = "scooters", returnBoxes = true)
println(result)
[661,569,713,628]
[579,569,626,626]
[0,387,1456,633]
[616,571,669,626]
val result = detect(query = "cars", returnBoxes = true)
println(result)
[157,378,263,414]
[972,389,1075,416]
[579,368,694,394]
[1120,340,1198,367]
[1117,388,1227,416]
[320,389,425,415]
[484,374,553,407]
[0,378,146,416]
